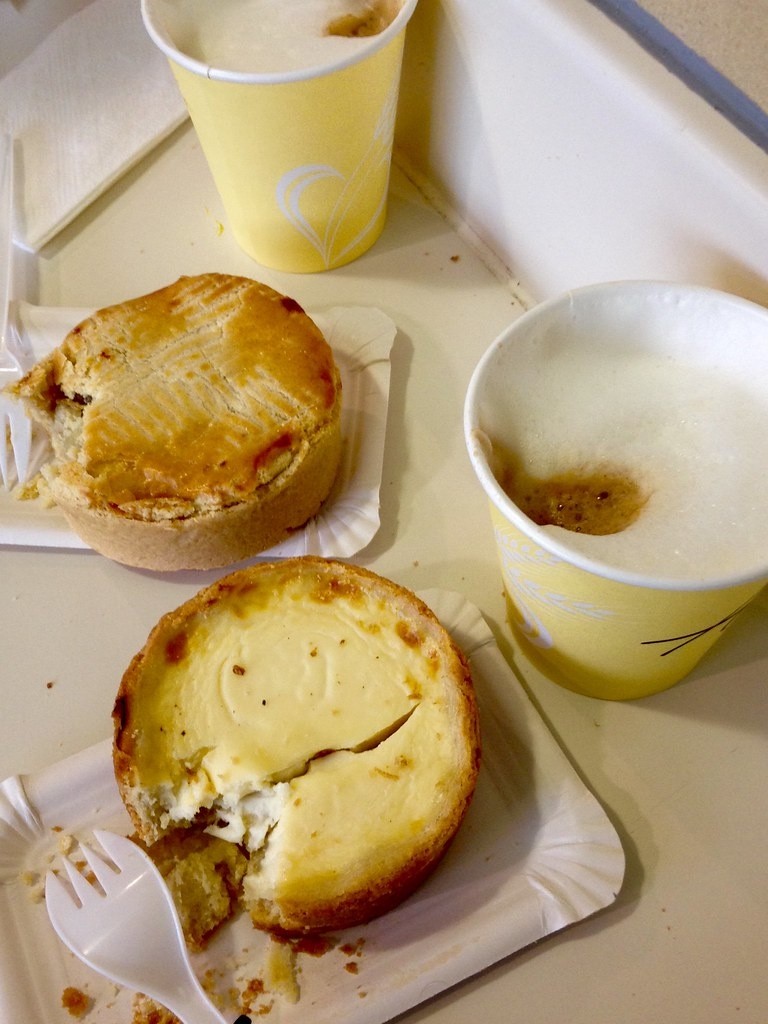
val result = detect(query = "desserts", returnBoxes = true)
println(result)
[6,273,346,573]
[110,557,481,949]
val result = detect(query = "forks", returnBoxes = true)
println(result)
[44,827,229,1024]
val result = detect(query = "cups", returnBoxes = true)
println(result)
[140,0,419,272]
[464,279,768,701]
